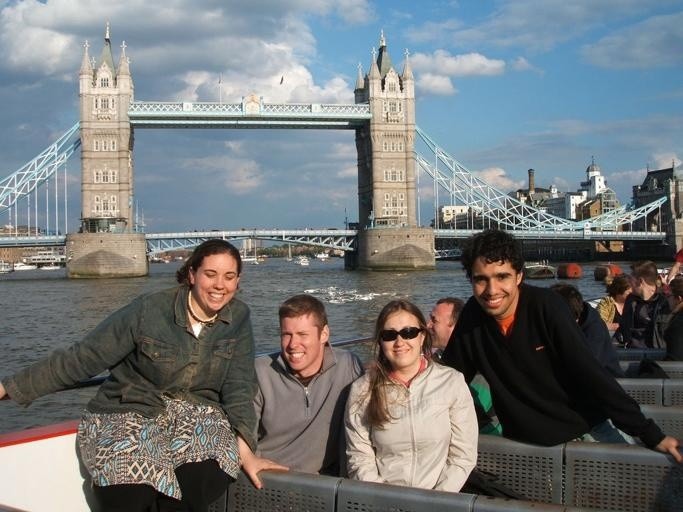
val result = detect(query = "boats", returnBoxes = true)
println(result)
[315,251,329,259]
[147,253,182,263]
[293,255,309,266]
[436,248,464,260]
[523,259,556,281]
[250,254,268,265]
[0,258,62,273]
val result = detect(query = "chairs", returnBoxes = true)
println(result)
[196,345,683,512]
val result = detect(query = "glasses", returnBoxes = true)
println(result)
[378,326,425,342]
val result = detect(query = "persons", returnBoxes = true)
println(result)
[427,296,504,436]
[596,246,683,361]
[254,294,366,475]
[549,281,624,379]
[440,227,683,464]
[1,239,289,512]
[342,300,478,494]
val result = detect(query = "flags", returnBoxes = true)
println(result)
[279,77,283,85]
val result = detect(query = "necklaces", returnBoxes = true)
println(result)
[187,290,219,325]
